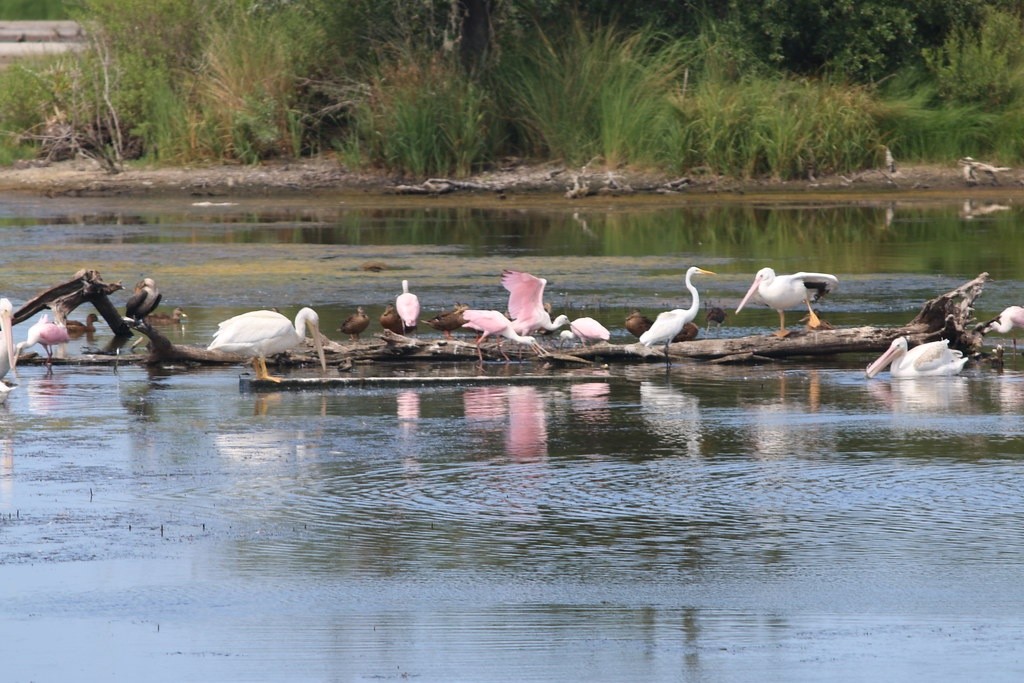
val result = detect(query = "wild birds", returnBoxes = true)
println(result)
[875,142,895,173]
[0,265,1024,406]
[958,156,1013,186]
